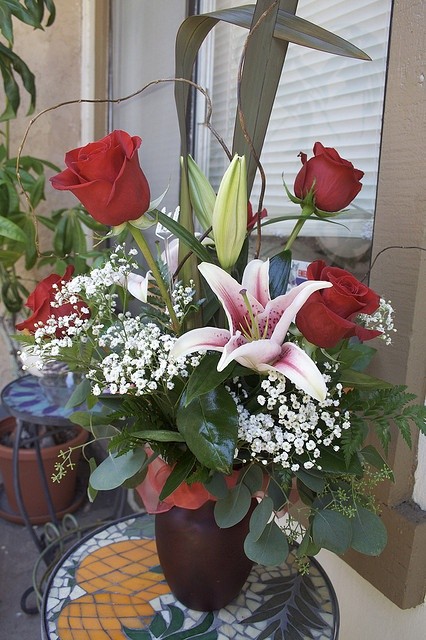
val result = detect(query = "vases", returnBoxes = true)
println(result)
[37,344,98,410]
[156,480,259,612]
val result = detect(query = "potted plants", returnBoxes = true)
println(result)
[0,0,111,527]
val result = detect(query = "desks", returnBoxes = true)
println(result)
[42,507,339,640]
[0,373,132,615]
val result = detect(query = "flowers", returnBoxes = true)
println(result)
[17,0,426,578]
